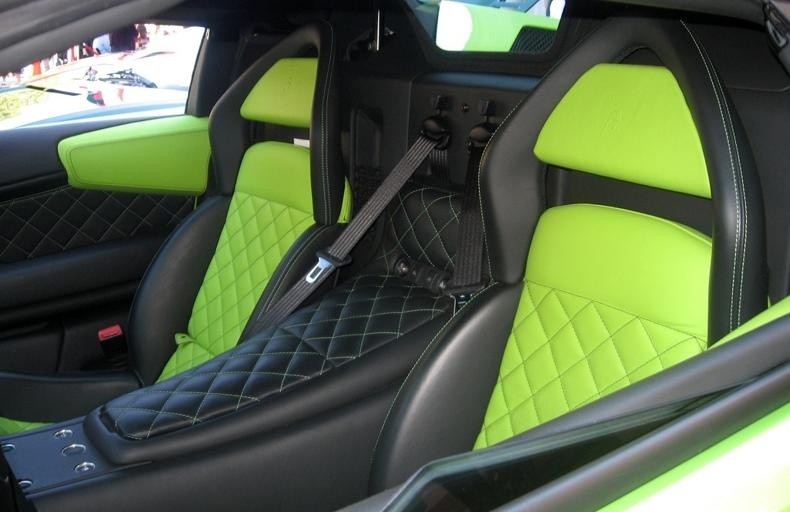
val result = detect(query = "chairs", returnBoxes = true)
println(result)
[0,16,355,440]
[370,14,790,511]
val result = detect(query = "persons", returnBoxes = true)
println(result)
[93,33,111,55]
[109,23,138,52]
